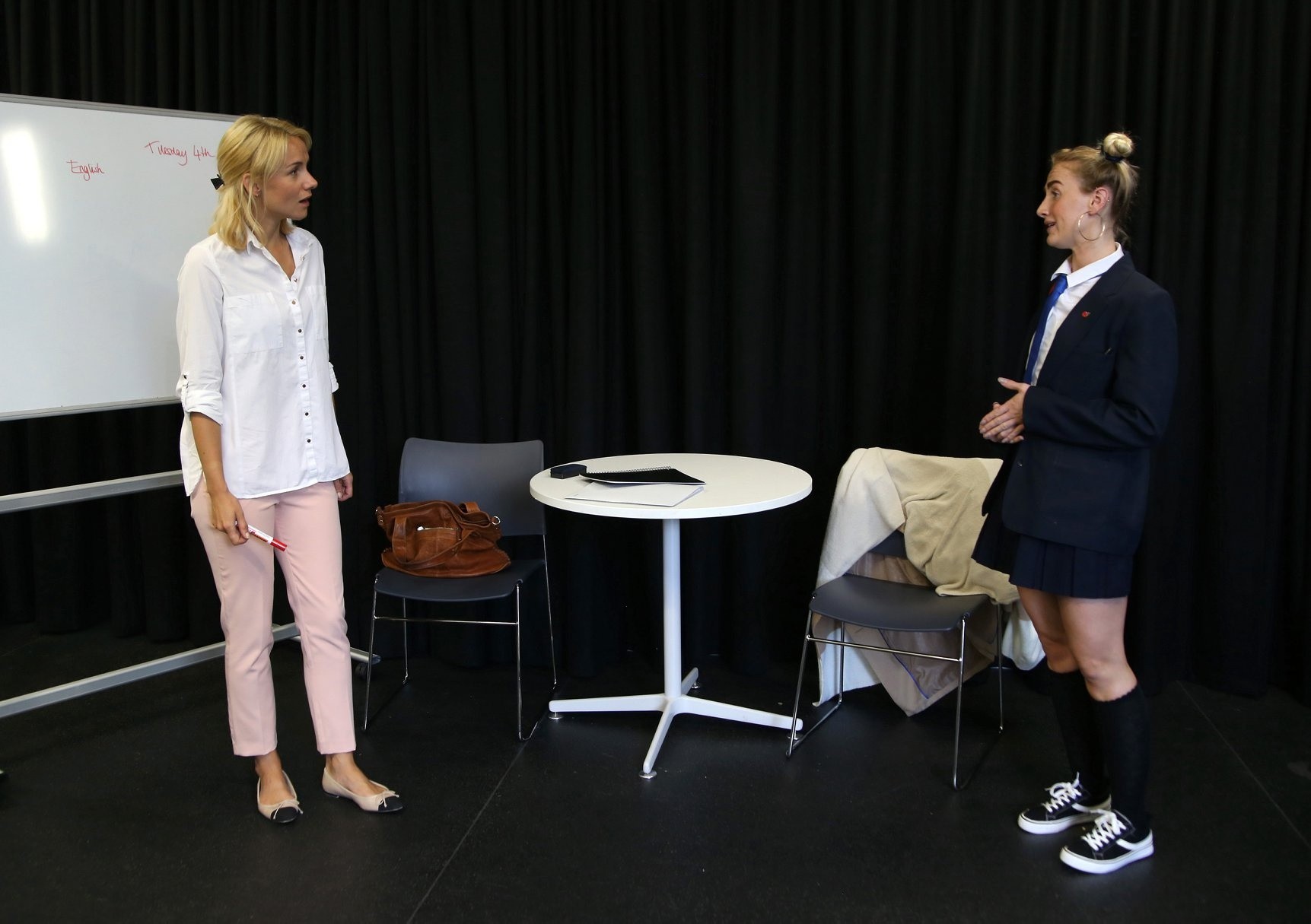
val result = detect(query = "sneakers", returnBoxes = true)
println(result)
[1017,772,1112,835]
[1059,809,1154,874]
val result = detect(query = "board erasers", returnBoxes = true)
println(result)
[550,464,587,480]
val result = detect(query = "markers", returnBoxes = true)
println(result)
[234,519,288,552]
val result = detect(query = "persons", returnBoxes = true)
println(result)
[972,133,1173,872]
[176,115,405,825]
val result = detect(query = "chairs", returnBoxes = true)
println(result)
[786,447,1020,793]
[362,438,557,743]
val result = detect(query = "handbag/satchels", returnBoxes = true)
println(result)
[375,500,511,577]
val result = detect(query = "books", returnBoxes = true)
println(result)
[578,466,706,484]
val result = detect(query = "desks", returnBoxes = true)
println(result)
[530,452,803,779]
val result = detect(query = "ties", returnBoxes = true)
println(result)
[1024,275,1068,385]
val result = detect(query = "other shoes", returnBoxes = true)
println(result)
[322,767,403,812]
[257,769,303,823]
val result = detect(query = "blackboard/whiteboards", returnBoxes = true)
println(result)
[0,92,242,422]
